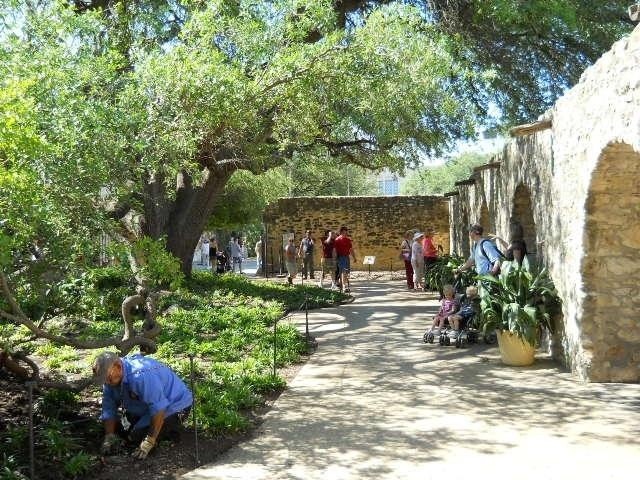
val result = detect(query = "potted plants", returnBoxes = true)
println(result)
[471,262,564,368]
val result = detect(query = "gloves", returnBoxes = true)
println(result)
[130,434,156,462]
[101,433,116,455]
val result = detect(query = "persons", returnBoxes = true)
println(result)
[90,351,193,460]
[283,238,297,286]
[446,286,482,338]
[427,283,459,335]
[487,233,530,327]
[206,234,263,275]
[411,232,425,291]
[399,229,415,289]
[422,227,438,290]
[297,225,356,293]
[455,223,503,330]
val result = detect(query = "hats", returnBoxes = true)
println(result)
[91,351,119,385]
[412,232,424,240]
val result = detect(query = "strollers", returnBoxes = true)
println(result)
[422,271,496,349]
[216,249,232,273]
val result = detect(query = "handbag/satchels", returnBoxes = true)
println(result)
[401,252,411,261]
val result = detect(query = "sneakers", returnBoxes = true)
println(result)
[449,330,460,339]
[425,329,441,336]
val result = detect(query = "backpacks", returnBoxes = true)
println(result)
[480,239,508,274]
